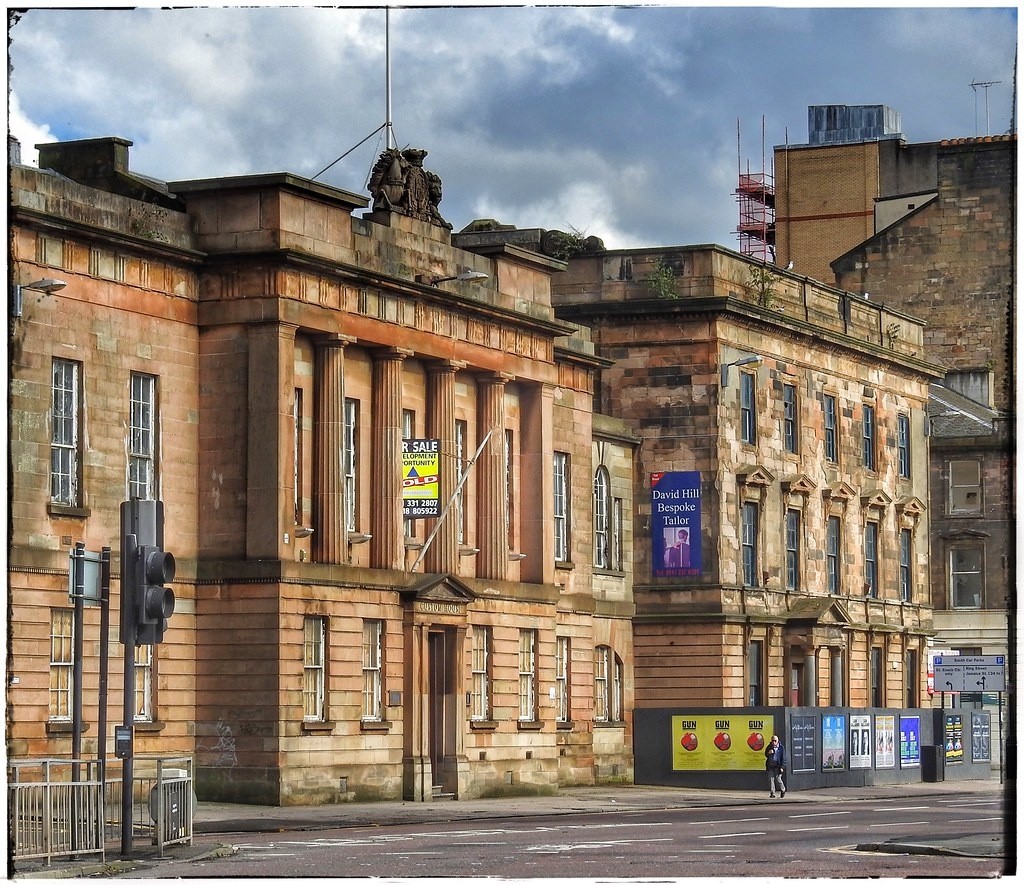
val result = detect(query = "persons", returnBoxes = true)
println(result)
[828,754,843,769]
[765,735,786,798]
[664,530,690,567]
[428,175,443,206]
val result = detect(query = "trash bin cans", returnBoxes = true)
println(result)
[921,745,944,782]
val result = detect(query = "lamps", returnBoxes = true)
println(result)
[14,278,67,316]
[429,269,489,286]
[720,356,763,387]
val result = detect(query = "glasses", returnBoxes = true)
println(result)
[772,740,778,743]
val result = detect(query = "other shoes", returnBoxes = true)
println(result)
[780,790,785,798]
[770,795,775,798]
